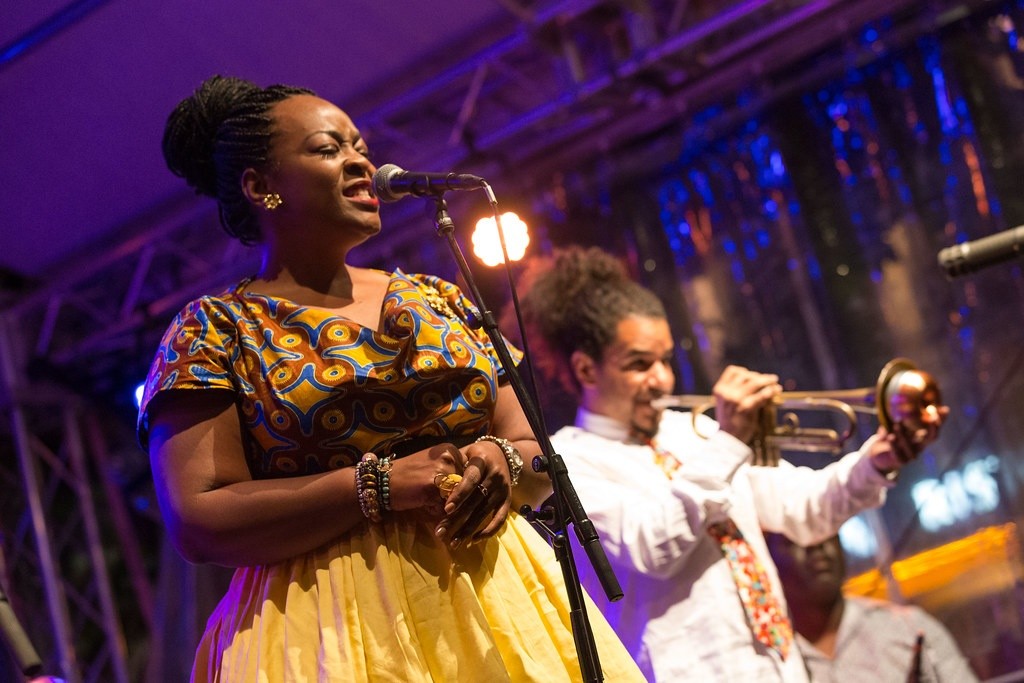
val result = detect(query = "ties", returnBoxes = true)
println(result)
[642,432,792,662]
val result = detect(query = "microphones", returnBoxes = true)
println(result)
[937,225,1024,282]
[371,164,487,203]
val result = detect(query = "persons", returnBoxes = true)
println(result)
[137,74,648,683]
[519,245,949,683]
[764,533,980,683]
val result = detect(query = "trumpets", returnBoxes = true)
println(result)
[654,357,943,469]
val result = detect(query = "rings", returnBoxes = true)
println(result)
[478,484,487,497]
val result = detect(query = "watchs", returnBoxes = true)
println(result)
[499,438,524,475]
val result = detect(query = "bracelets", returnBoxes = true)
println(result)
[475,435,518,487]
[355,453,392,523]
